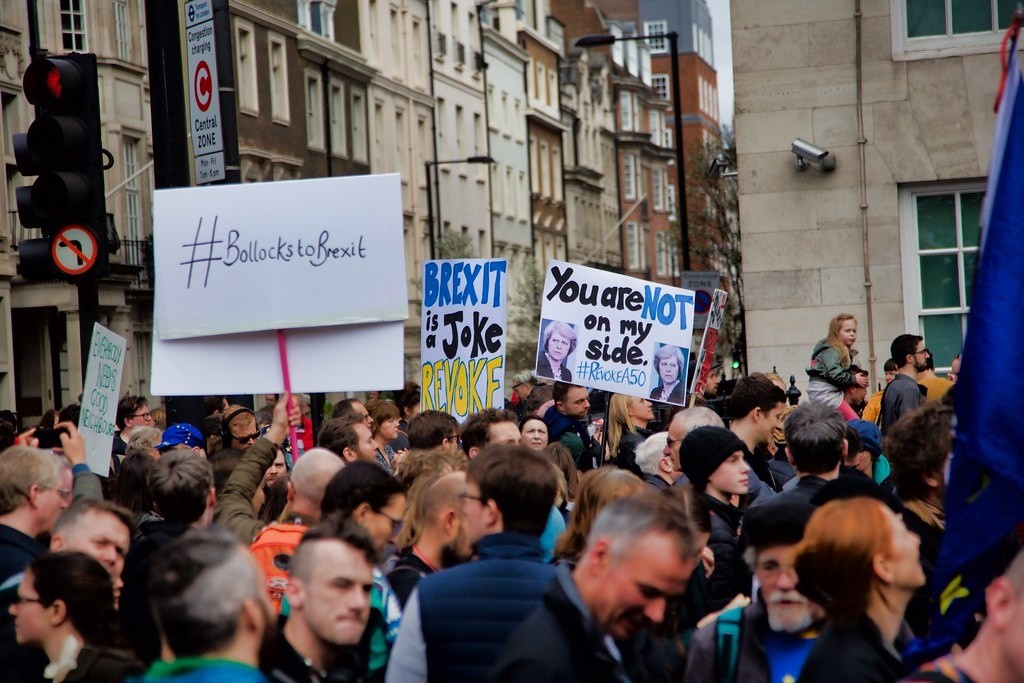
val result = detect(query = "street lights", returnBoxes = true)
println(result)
[576,32,691,275]
[424,157,494,258]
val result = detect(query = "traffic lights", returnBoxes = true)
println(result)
[23,53,107,235]
[12,131,78,283]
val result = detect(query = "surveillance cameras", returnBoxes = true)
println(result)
[708,154,729,177]
[791,139,829,163]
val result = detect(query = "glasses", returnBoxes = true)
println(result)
[36,482,71,501]
[15,591,51,607]
[445,434,462,444]
[231,427,260,444]
[130,412,151,421]
[458,492,485,506]
[666,437,683,448]
[911,348,926,358]
[368,506,402,538]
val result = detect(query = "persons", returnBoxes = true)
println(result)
[8,552,145,683]
[485,493,701,683]
[384,442,561,683]
[0,500,137,611]
[900,549,1024,682]
[684,522,827,683]
[258,529,394,683]
[780,497,927,683]
[125,526,280,683]
[650,346,685,404]
[807,313,868,422]
[879,333,930,438]
[0,347,958,673]
[537,320,577,383]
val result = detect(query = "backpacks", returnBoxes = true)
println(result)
[247,521,312,619]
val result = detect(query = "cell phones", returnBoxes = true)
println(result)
[33,428,70,448]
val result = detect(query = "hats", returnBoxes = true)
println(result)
[153,423,202,450]
[739,488,821,552]
[678,426,746,488]
[848,418,883,462]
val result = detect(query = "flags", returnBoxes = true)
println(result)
[927,39,1023,604]
